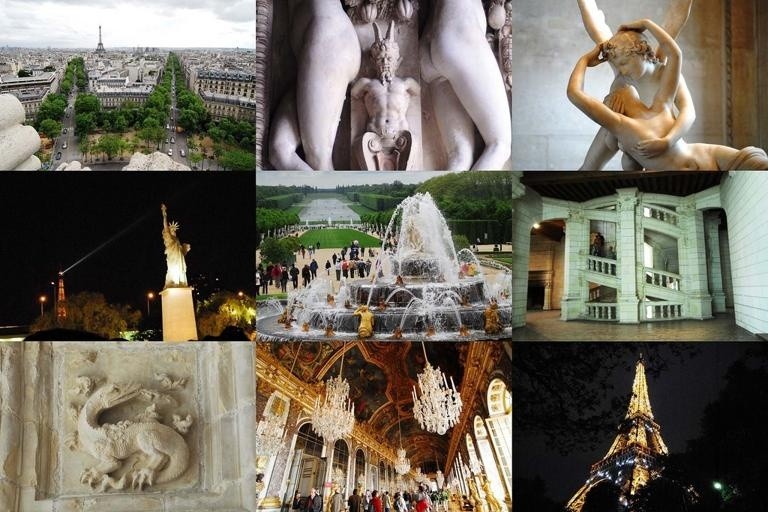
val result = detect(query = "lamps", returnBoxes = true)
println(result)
[311,340,464,490]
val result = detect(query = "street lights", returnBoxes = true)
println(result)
[238,291,244,300]
[147,293,153,318]
[51,282,57,317]
[39,296,46,316]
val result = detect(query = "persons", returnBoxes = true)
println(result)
[460,494,474,511]
[566,18,768,170]
[466,260,477,275]
[418,0,513,171]
[161,203,191,290]
[460,260,469,274]
[471,243,480,253]
[270,1,361,169]
[255,473,264,501]
[593,235,602,256]
[604,245,615,274]
[295,484,433,512]
[484,301,502,333]
[350,21,422,171]
[575,0,693,170]
[492,244,498,252]
[597,232,604,247]
[256,240,382,294]
[353,303,376,337]
[360,224,398,258]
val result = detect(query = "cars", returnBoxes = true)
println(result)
[165,73,176,158]
[132,326,163,341]
[55,88,75,161]
[180,148,186,157]
